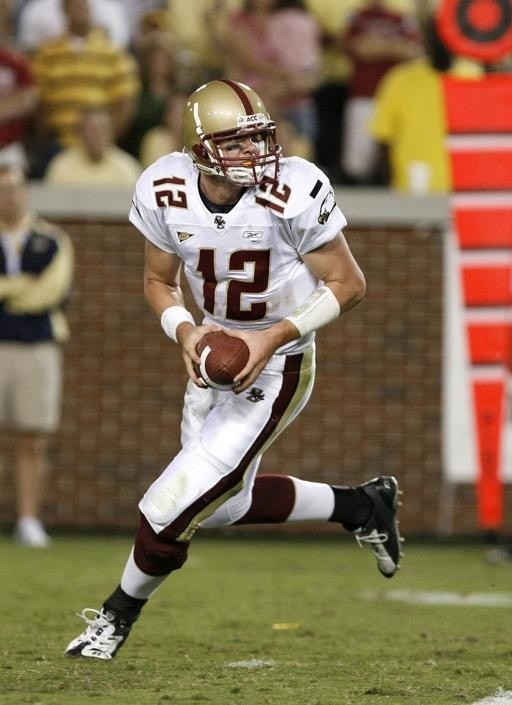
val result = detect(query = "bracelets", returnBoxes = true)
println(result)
[160,306,195,345]
[284,286,341,337]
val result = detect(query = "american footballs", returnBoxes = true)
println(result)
[192,332,250,391]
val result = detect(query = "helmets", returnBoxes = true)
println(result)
[181,77,283,188]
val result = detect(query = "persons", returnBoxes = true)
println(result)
[2,1,340,193]
[344,1,426,184]
[0,164,75,550]
[64,79,406,663]
[371,1,486,192]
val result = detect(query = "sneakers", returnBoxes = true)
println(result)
[16,518,51,548]
[345,474,403,579]
[63,604,132,661]
